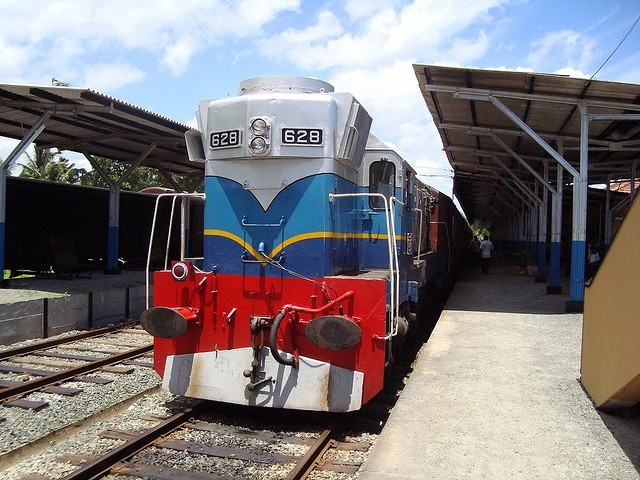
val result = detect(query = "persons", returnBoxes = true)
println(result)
[470,235,480,252]
[480,235,493,272]
[588,244,600,265]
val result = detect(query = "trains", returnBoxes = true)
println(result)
[139,74,476,428]
